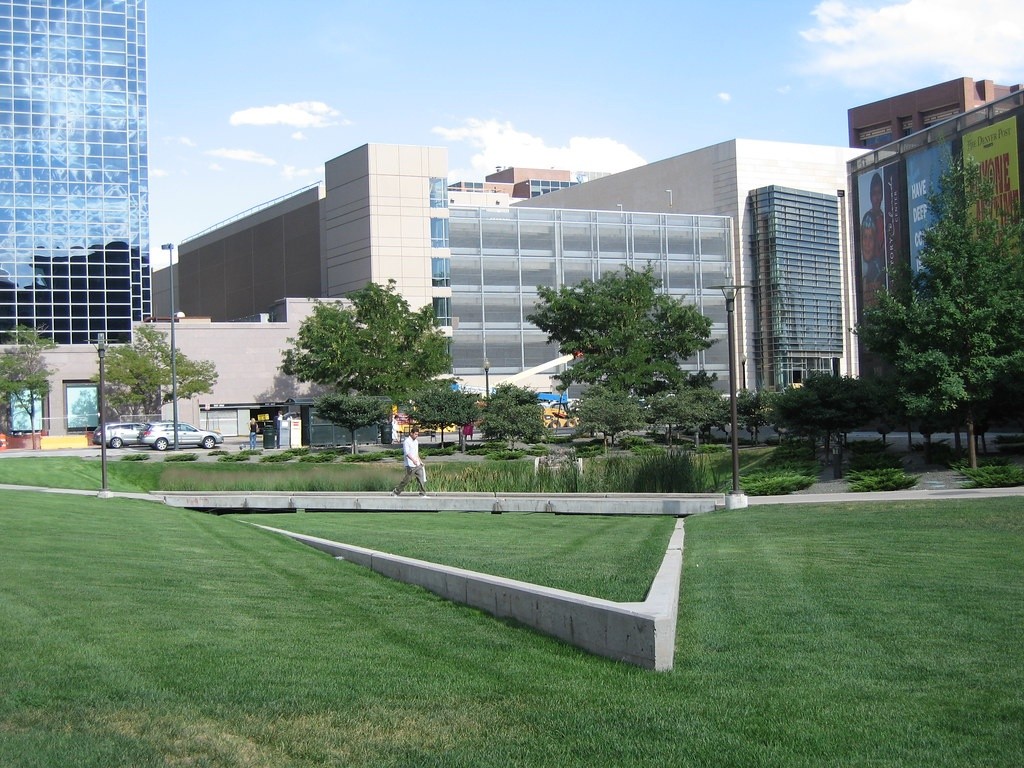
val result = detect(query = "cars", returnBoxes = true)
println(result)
[137,421,224,450]
[93,422,144,449]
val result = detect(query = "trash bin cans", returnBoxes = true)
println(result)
[262,427,275,449]
[381,424,392,444]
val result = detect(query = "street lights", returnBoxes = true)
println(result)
[84,333,118,499]
[705,275,753,494]
[169,243,186,451]
[483,358,490,407]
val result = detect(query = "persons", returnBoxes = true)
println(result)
[249,418,258,449]
[392,415,400,444]
[390,428,426,496]
[463,422,473,440]
[861,173,886,287]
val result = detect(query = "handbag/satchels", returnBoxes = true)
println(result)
[418,464,427,482]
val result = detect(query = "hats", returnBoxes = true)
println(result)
[394,415,400,418]
